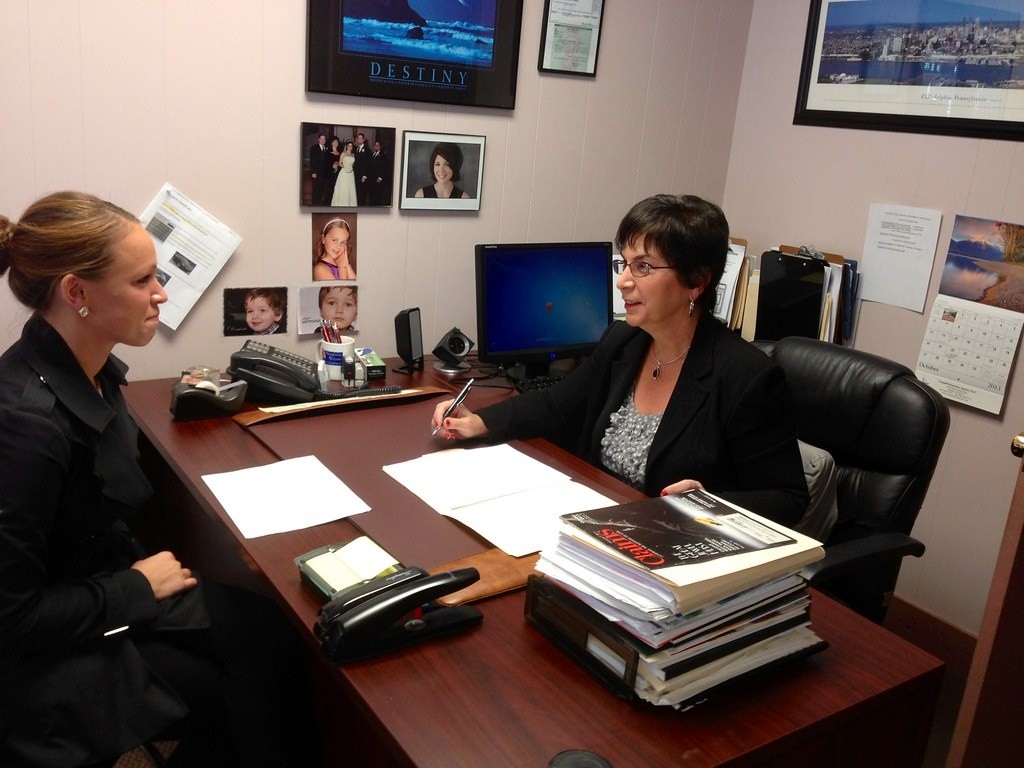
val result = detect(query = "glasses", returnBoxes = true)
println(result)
[613,259,672,276]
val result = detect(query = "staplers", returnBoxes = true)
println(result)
[313,567,486,667]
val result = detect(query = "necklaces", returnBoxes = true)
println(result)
[651,343,691,381]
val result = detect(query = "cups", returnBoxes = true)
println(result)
[316,336,355,381]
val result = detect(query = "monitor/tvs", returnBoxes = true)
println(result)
[476,241,613,383]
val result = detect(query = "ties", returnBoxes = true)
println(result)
[358,147,360,154]
[373,151,379,156]
[322,146,324,152]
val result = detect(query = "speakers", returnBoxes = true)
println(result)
[391,307,424,375]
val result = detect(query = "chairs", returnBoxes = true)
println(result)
[749,336,950,626]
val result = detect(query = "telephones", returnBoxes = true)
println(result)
[225,338,320,402]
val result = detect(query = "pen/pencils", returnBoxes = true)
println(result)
[430,377,475,436]
[319,315,342,344]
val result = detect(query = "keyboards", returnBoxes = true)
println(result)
[515,374,564,392]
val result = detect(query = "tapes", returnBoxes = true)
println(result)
[195,380,247,397]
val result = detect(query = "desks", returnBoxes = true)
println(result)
[121,350,942,768]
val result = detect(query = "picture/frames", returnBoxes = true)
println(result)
[303,0,524,110]
[793,0,1024,142]
[299,122,396,208]
[399,130,486,210]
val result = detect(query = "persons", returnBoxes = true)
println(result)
[312,217,357,280]
[430,193,810,526]
[0,191,321,768]
[310,133,393,208]
[244,288,285,335]
[414,142,470,200]
[315,286,358,334]
